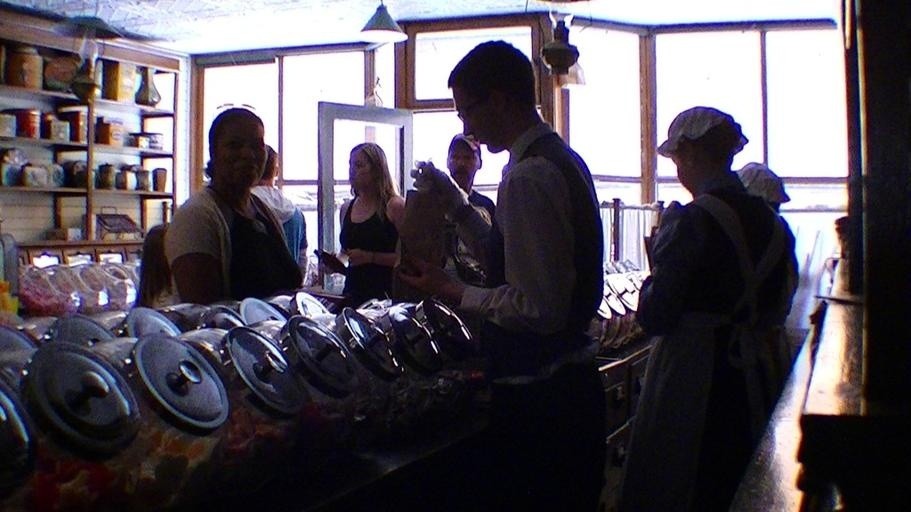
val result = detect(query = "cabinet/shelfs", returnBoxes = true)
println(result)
[2,3,179,295]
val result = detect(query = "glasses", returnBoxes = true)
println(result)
[457,99,482,121]
[216,102,258,110]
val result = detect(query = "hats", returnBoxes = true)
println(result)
[733,162,791,202]
[448,133,482,156]
[655,106,749,158]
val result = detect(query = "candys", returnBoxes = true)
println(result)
[18,275,126,316]
[587,313,639,346]
[0,372,485,512]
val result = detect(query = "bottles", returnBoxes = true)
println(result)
[136,67,162,103]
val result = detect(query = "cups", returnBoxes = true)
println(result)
[99,162,116,189]
[153,167,167,192]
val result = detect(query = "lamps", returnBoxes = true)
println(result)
[534,0,587,90]
[53,0,120,67]
[360,0,409,46]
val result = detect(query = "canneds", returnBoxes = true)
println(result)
[19,109,42,138]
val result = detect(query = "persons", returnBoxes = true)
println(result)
[163,107,307,306]
[637,105,799,512]
[319,144,404,307]
[399,41,606,511]
[132,223,181,311]
